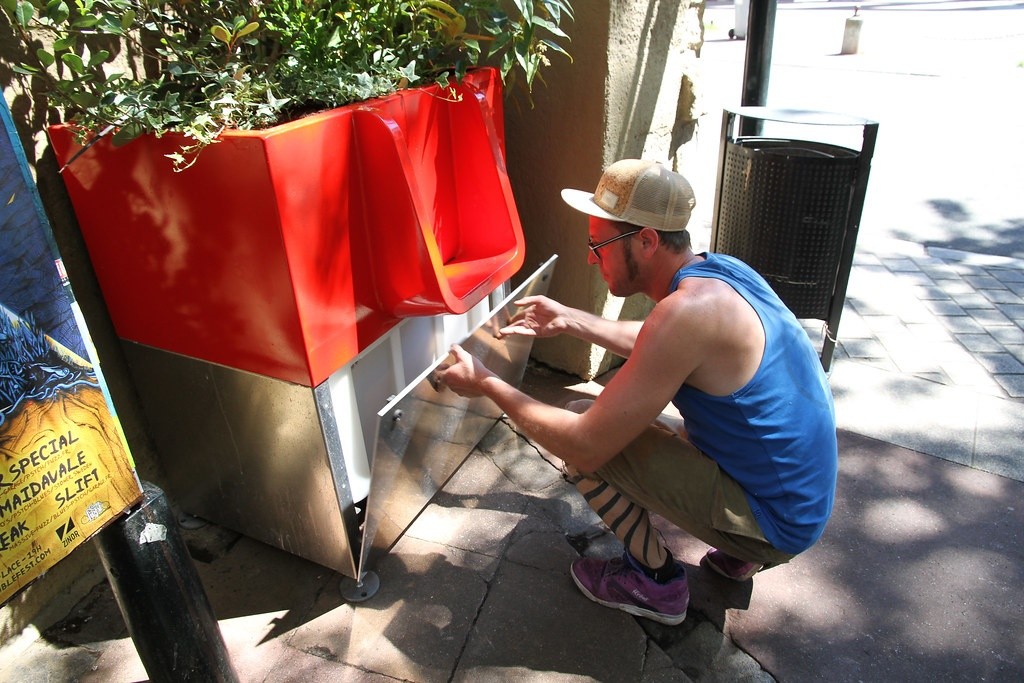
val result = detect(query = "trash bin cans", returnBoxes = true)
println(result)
[709,107,879,372]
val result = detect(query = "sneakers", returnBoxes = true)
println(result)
[570,551,690,625]
[706,544,761,582]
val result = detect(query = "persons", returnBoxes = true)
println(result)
[426,159,839,628]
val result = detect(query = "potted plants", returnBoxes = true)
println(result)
[0,1,575,386]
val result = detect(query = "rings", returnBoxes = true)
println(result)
[437,377,442,384]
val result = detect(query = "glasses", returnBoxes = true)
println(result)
[587,229,641,259]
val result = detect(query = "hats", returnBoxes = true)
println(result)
[560,158,697,232]
